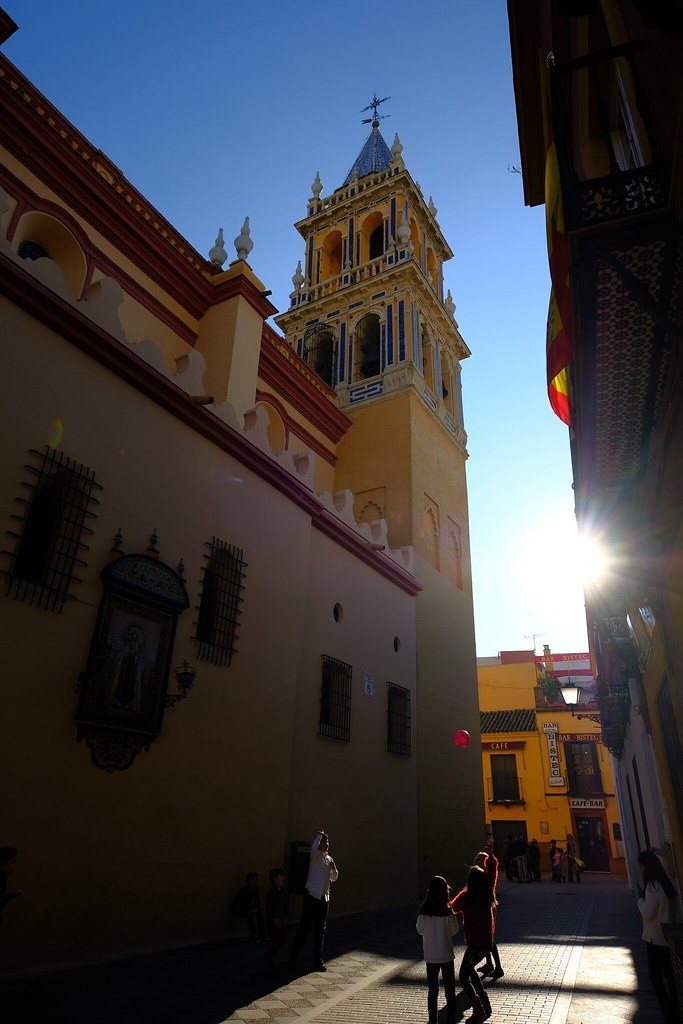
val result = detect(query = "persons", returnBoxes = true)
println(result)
[266,869,292,942]
[303,825,339,971]
[449,866,492,1024]
[233,873,268,944]
[0,847,23,923]
[506,833,542,883]
[473,835,503,978]
[549,841,566,883]
[416,875,460,1024]
[636,850,680,1023]
[566,834,581,883]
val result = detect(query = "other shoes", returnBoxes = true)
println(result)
[477,963,494,972]
[312,964,326,971]
[259,935,270,941]
[489,968,504,977]
[250,937,261,943]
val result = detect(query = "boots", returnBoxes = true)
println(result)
[480,993,492,1013]
[465,996,486,1024]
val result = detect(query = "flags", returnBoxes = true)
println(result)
[538,52,575,426]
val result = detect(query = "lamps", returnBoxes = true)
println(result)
[556,676,629,728]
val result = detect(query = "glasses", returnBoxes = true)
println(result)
[251,877,259,880]
[476,858,483,861]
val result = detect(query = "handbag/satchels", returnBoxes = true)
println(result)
[574,856,585,868]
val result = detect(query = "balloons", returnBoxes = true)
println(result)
[454,729,471,749]
